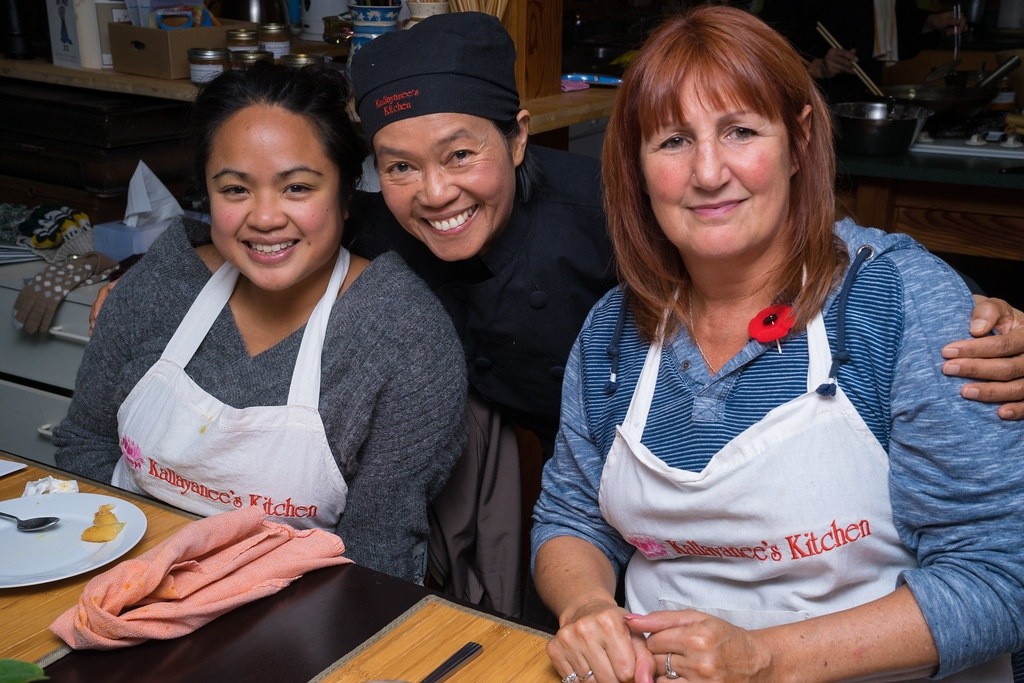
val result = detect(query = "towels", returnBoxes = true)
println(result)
[44,504,355,652]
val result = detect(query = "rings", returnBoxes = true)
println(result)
[580,670,593,682]
[665,652,679,679]
[562,672,576,683]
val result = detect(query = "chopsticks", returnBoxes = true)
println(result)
[953,4,962,63]
[815,21,886,98]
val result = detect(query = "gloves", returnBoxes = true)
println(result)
[9,253,120,338]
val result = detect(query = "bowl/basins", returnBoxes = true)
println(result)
[827,99,933,159]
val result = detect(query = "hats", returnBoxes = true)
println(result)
[350,14,521,155]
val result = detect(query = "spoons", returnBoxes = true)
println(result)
[0,512,60,531]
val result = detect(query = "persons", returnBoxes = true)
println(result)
[53,10,471,682]
[87,12,1023,635]
[532,4,1021,683]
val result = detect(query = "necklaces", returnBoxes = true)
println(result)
[687,284,717,373]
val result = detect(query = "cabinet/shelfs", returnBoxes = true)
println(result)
[0,1,621,461]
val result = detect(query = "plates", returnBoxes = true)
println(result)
[0,494,147,589]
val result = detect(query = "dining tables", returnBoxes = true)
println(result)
[1,449,568,683]
[826,49,1023,271]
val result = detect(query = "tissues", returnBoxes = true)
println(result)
[92,158,217,265]
